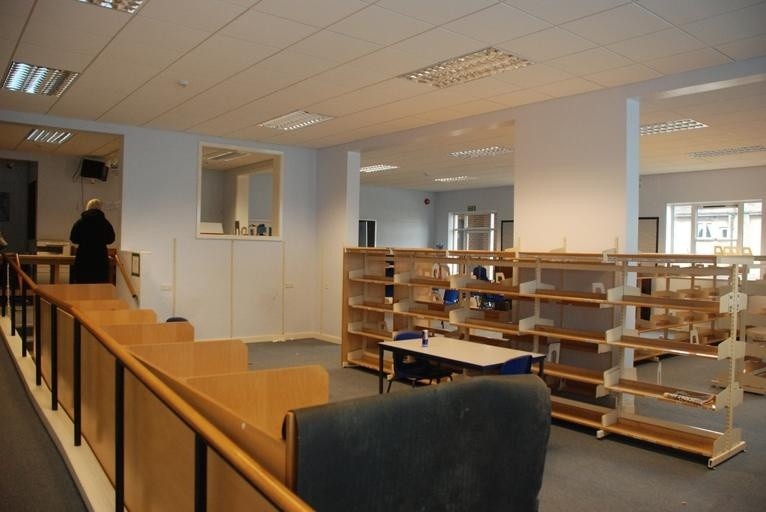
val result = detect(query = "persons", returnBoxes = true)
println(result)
[69,199,115,284]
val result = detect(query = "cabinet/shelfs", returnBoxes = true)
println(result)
[337,246,765,468]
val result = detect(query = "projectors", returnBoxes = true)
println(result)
[479,297,512,311]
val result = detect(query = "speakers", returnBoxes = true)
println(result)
[101,166,109,181]
[358,220,375,247]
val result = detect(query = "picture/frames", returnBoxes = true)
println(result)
[639,217,659,267]
[500,220,515,251]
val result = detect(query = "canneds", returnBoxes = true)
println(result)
[421,328,429,347]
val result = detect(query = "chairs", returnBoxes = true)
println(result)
[38,283,552,512]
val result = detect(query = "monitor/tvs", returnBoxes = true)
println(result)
[80,158,104,178]
